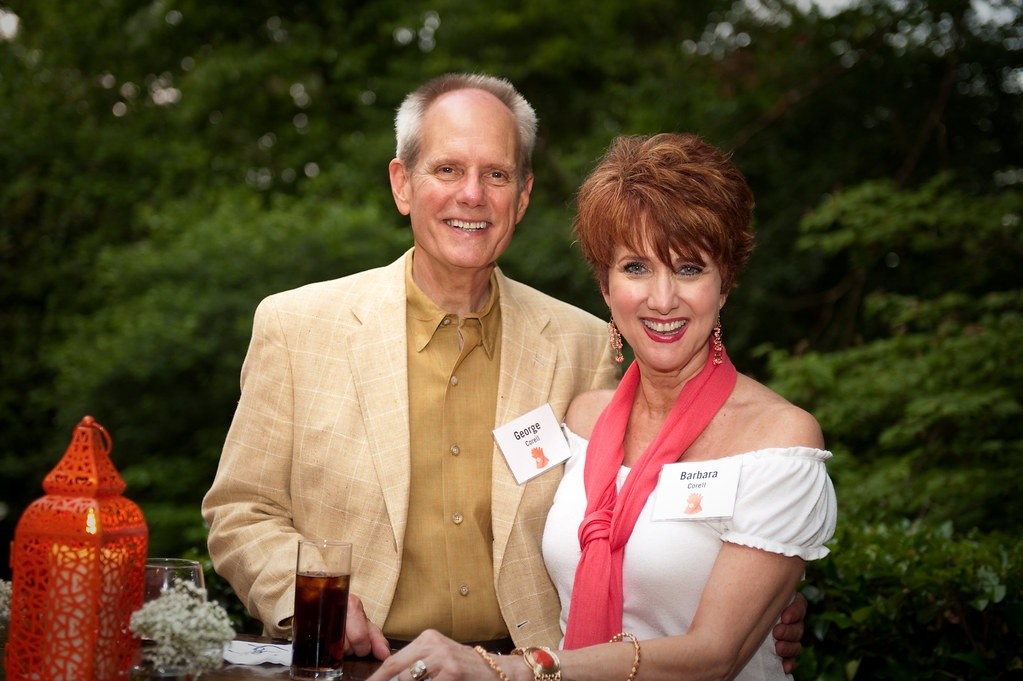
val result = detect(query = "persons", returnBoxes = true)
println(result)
[200,72,809,674]
[363,133,838,681]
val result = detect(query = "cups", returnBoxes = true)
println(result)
[144,558,205,603]
[289,540,353,681]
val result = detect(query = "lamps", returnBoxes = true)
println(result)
[0,414,150,681]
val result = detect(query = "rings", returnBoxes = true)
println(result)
[408,661,428,681]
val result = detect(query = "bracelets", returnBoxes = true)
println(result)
[474,645,511,681]
[511,646,561,681]
[609,632,642,681]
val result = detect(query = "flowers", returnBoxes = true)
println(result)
[131,578,236,681]
